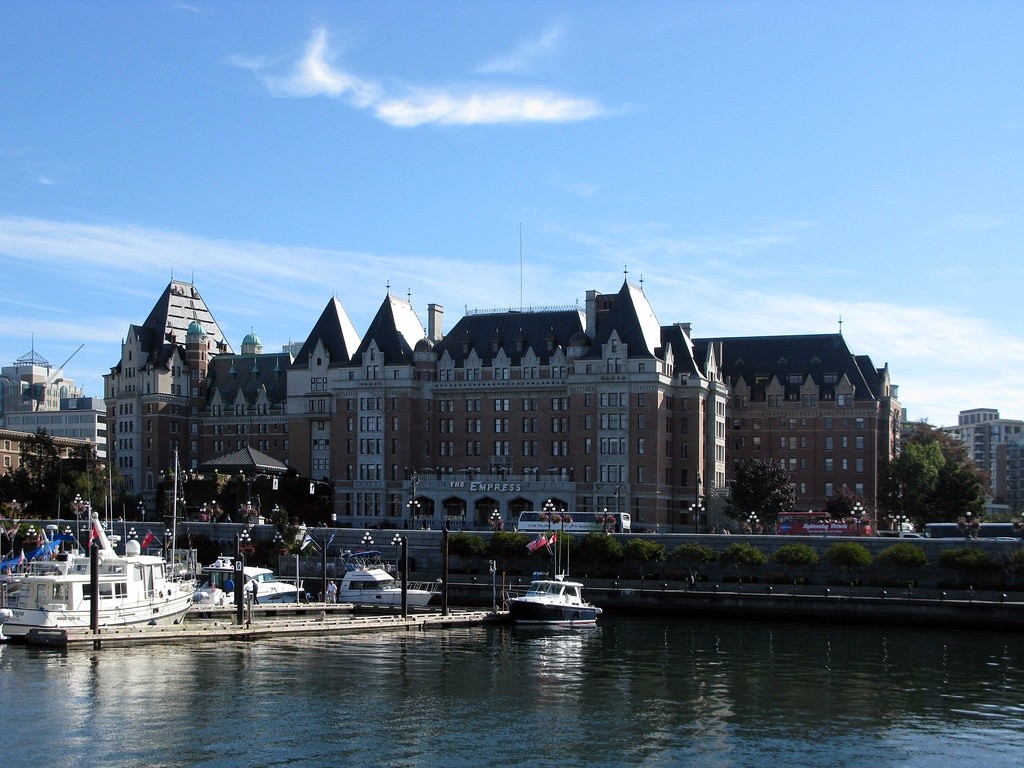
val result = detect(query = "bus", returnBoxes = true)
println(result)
[516,511,632,533]
[776,510,871,536]
[926,522,1016,537]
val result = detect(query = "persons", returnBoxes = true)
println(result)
[224,577,234,593]
[328,581,337,604]
[252,580,259,604]
[381,562,391,572]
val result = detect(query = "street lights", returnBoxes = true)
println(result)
[614,481,621,513]
[965,511,972,539]
[893,515,910,537]
[73,494,83,553]
[560,508,565,532]
[543,498,556,532]
[1020,511,1024,523]
[272,530,284,575]
[407,464,421,530]
[491,509,501,532]
[164,529,172,564]
[850,502,865,536]
[360,531,374,553]
[239,529,252,565]
[603,507,608,532]
[747,511,760,536]
[689,467,706,533]
[390,533,402,580]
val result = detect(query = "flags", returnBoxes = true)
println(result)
[526,533,556,551]
[88,528,98,548]
[300,534,312,550]
[141,530,154,548]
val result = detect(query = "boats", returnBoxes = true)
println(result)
[506,531,603,625]
[0,451,442,640]
